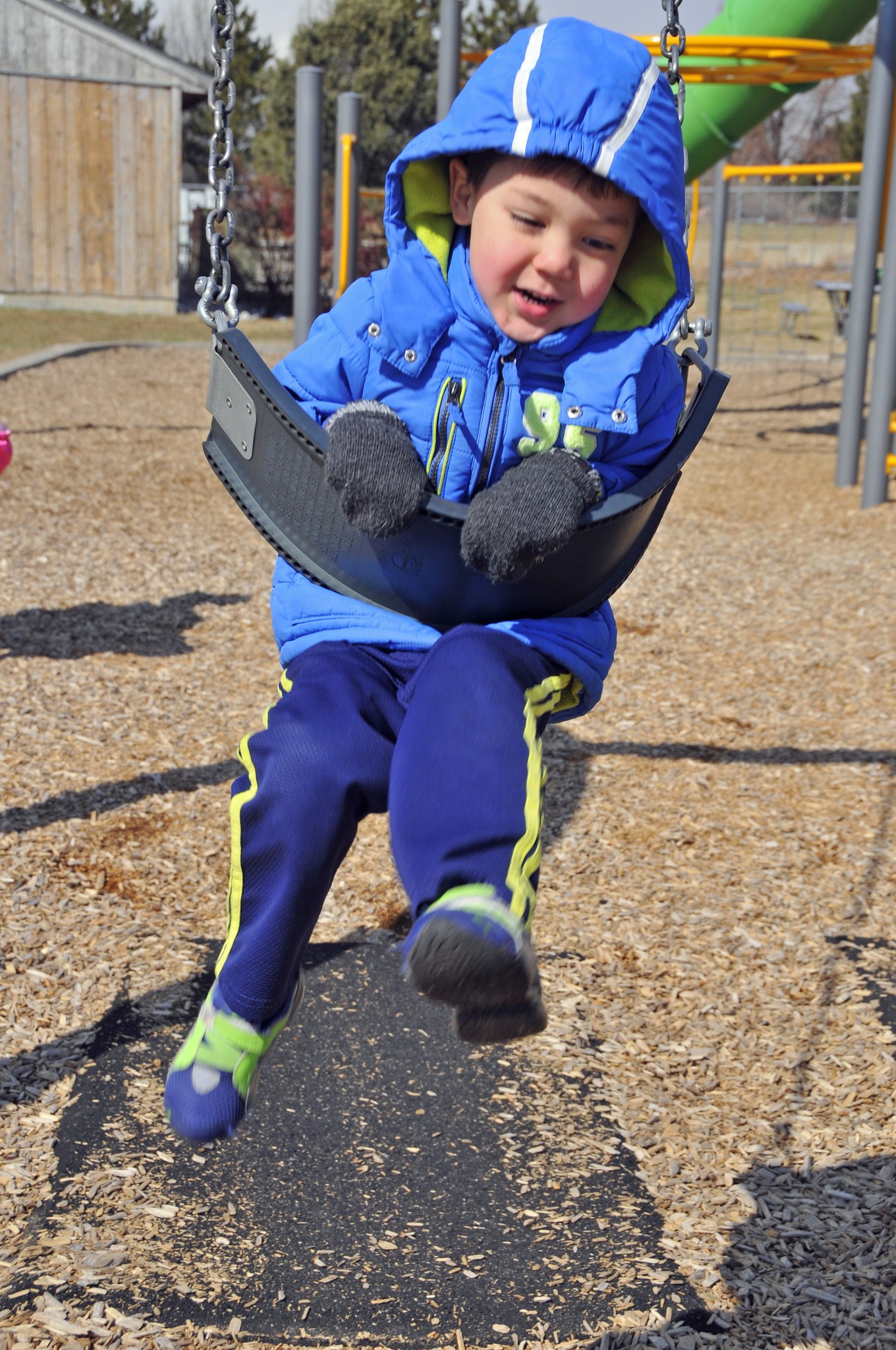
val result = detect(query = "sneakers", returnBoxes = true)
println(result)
[164,967,305,1140]
[401,884,548,1044]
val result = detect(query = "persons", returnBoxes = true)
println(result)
[163,17,691,1144]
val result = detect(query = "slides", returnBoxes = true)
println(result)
[655,1,879,190]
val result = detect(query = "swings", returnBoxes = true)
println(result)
[195,1,733,631]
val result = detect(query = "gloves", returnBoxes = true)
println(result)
[460,450,594,584]
[326,397,427,539]
